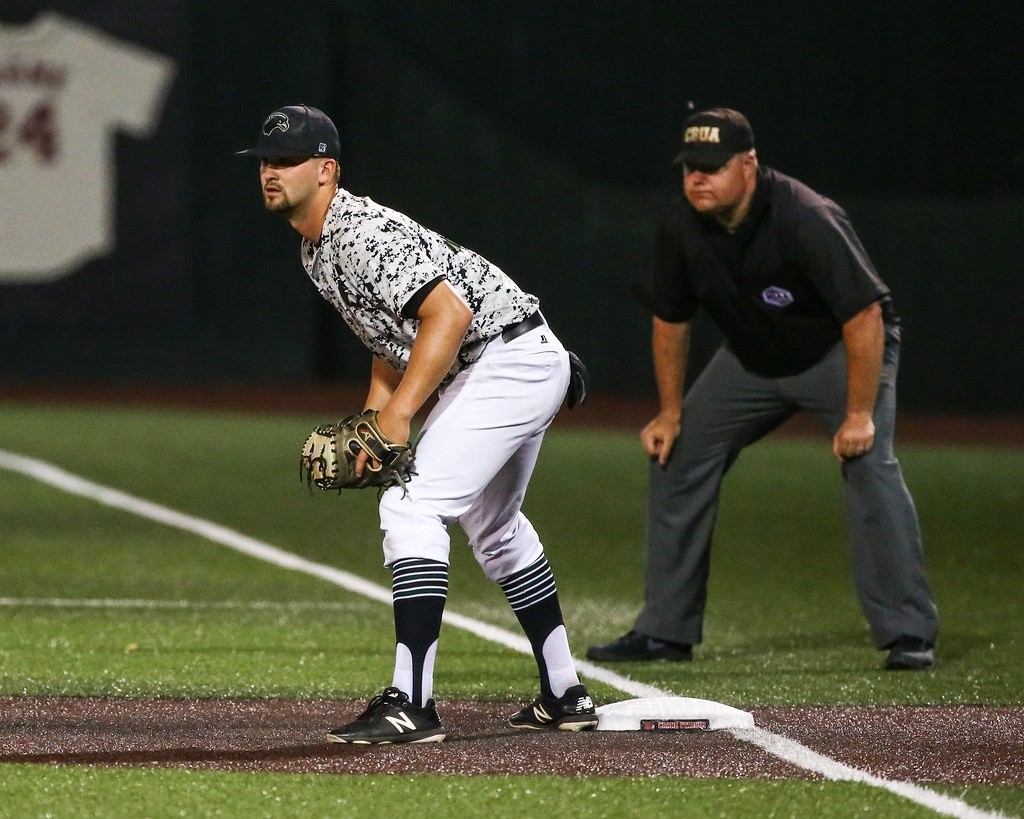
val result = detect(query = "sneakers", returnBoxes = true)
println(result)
[507,685,599,732]
[886,639,934,668]
[587,630,693,662]
[327,687,447,744]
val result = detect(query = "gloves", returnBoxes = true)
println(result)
[567,351,590,410]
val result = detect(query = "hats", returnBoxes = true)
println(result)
[673,108,754,166]
[233,103,341,161]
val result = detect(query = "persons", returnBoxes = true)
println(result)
[233,104,601,745]
[585,108,939,670]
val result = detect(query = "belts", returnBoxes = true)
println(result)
[502,311,544,344]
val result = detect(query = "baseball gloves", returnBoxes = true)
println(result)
[300,407,414,496]
